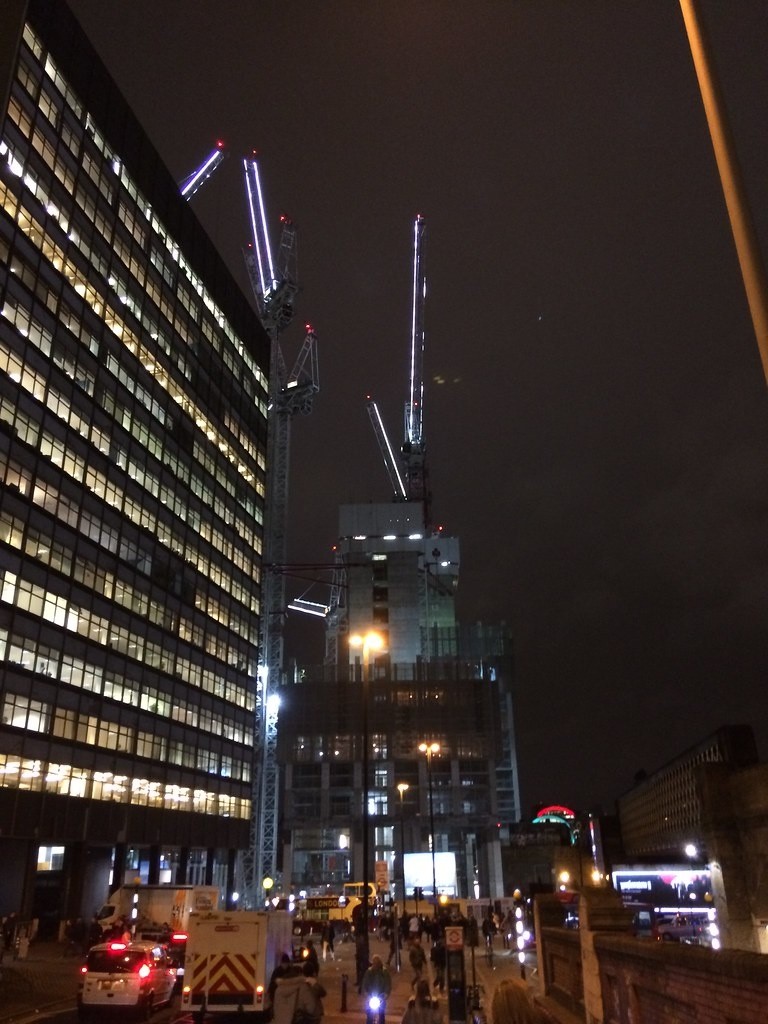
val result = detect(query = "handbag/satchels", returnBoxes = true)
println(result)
[290,988,315,1024]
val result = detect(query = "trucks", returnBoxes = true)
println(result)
[181,912,293,1024]
[94,885,219,941]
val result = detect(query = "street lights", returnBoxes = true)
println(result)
[397,784,409,911]
[572,828,584,890]
[704,892,713,910]
[419,744,440,919]
[349,634,384,973]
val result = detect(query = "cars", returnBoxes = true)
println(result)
[77,941,177,1021]
[658,916,705,942]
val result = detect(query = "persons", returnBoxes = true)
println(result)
[400,980,442,1024]
[410,936,426,990]
[321,909,517,951]
[63,916,102,955]
[266,940,328,1024]
[491,979,561,1024]
[3,911,17,948]
[385,927,403,967]
[430,940,446,990]
[360,955,392,1023]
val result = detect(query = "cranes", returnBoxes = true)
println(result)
[184,144,430,912]
